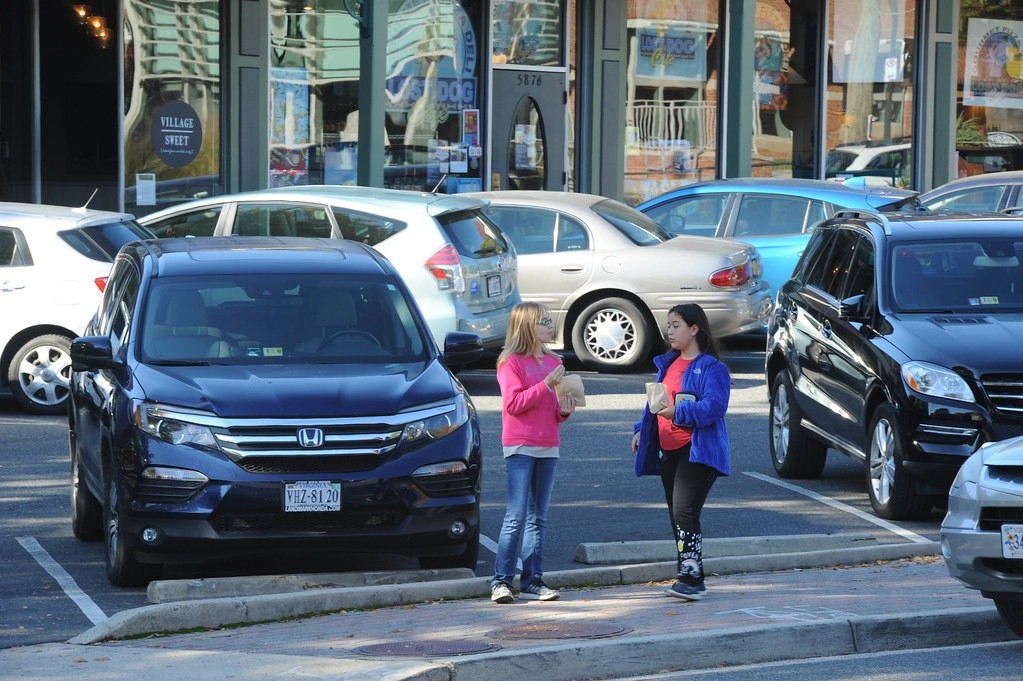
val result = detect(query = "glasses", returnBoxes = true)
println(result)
[537,318,553,326]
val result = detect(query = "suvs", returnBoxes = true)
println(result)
[765,227,1023,522]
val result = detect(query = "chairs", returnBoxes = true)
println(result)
[158,290,208,335]
[895,257,927,307]
[295,288,367,351]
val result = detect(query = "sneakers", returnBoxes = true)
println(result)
[492,583,513,601]
[518,578,560,600]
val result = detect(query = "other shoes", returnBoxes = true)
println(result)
[666,583,707,600]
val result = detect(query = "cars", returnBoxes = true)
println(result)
[353,188,776,375]
[65,235,487,588]
[536,178,954,347]
[0,201,183,417]
[826,139,1022,194]
[136,184,520,379]
[903,170,1022,272]
[939,436,1023,638]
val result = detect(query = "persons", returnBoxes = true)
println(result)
[632,302,731,599]
[490,301,576,601]
[753,31,784,138]
